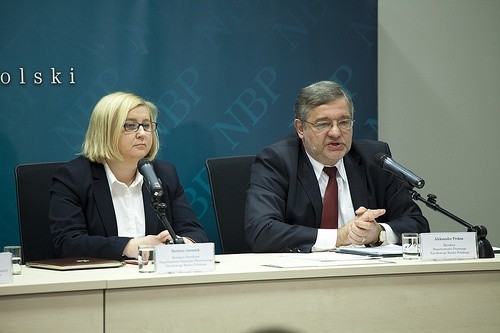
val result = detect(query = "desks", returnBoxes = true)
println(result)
[0,251,500,333]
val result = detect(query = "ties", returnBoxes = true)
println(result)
[321,166,338,229]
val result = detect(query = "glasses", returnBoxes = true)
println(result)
[300,119,355,133]
[124,122,157,132]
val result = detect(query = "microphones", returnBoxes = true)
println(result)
[138,158,163,201]
[374,153,425,188]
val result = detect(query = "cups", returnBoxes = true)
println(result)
[4,246,22,275]
[402,233,419,260]
[138,246,155,272]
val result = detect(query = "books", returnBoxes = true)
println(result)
[26,256,127,270]
[335,243,403,257]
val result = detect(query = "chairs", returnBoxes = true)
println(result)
[15,162,65,264]
[206,156,254,253]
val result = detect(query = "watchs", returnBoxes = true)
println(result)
[369,224,386,247]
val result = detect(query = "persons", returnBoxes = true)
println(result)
[44,91,210,261]
[238,80,430,253]
